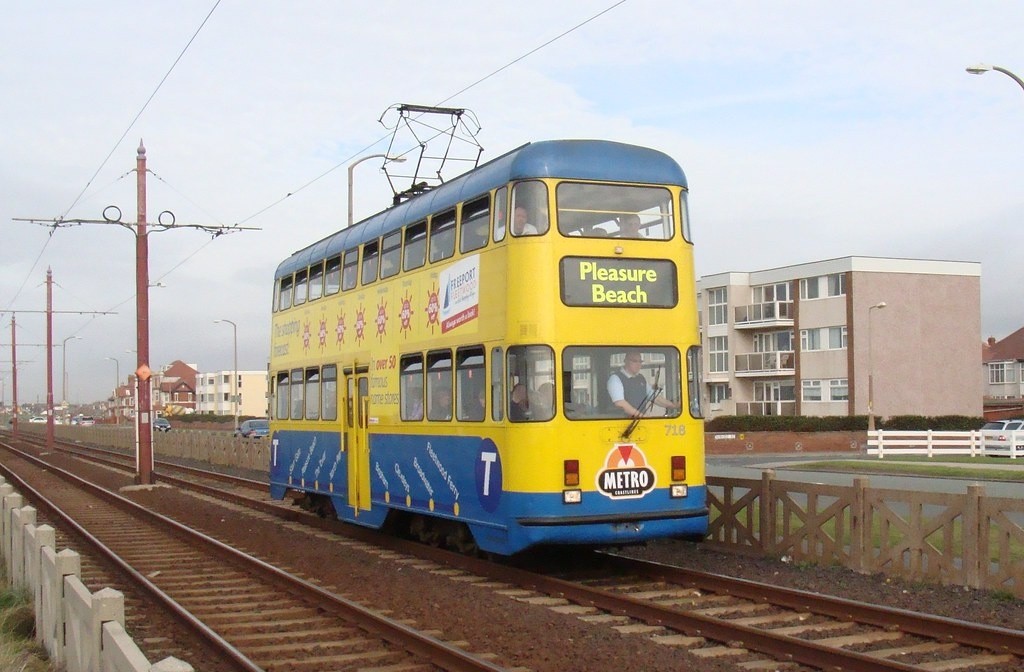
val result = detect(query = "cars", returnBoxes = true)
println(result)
[152,417,172,433]
[976,419,1024,459]
[53,413,95,428]
[29,416,47,425]
[233,419,269,440]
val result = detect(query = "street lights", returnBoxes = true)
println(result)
[105,356,120,423]
[868,302,889,440]
[214,319,238,428]
[62,335,84,410]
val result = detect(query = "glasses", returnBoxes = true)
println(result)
[630,359,643,364]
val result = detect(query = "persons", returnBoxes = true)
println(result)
[606,352,681,417]
[510,383,553,420]
[496,205,537,240]
[621,214,644,237]
[407,386,486,420]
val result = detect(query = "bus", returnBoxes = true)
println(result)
[266,102,711,567]
